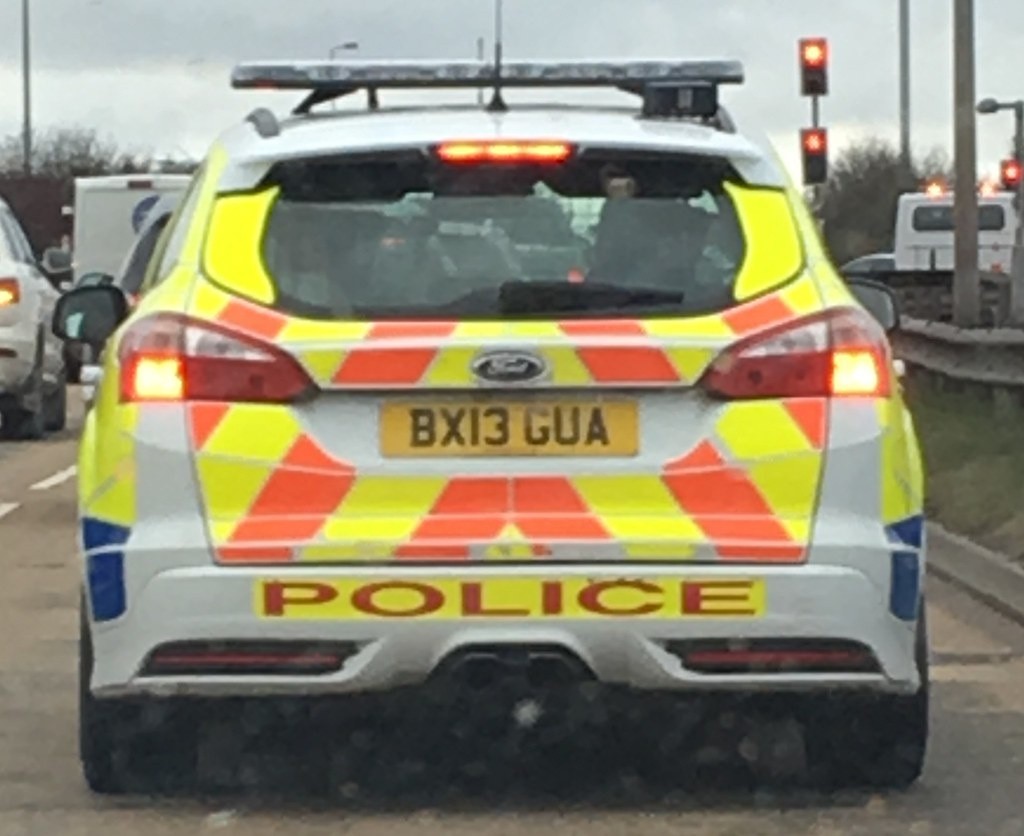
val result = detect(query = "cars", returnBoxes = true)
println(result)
[0,192,68,434]
[54,53,935,801]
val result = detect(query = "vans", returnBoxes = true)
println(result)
[890,189,1017,274]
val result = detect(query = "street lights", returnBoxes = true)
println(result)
[976,96,1024,161]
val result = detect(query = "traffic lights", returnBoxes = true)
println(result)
[800,127,829,187]
[800,36,827,95]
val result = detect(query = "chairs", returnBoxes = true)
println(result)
[589,197,709,286]
